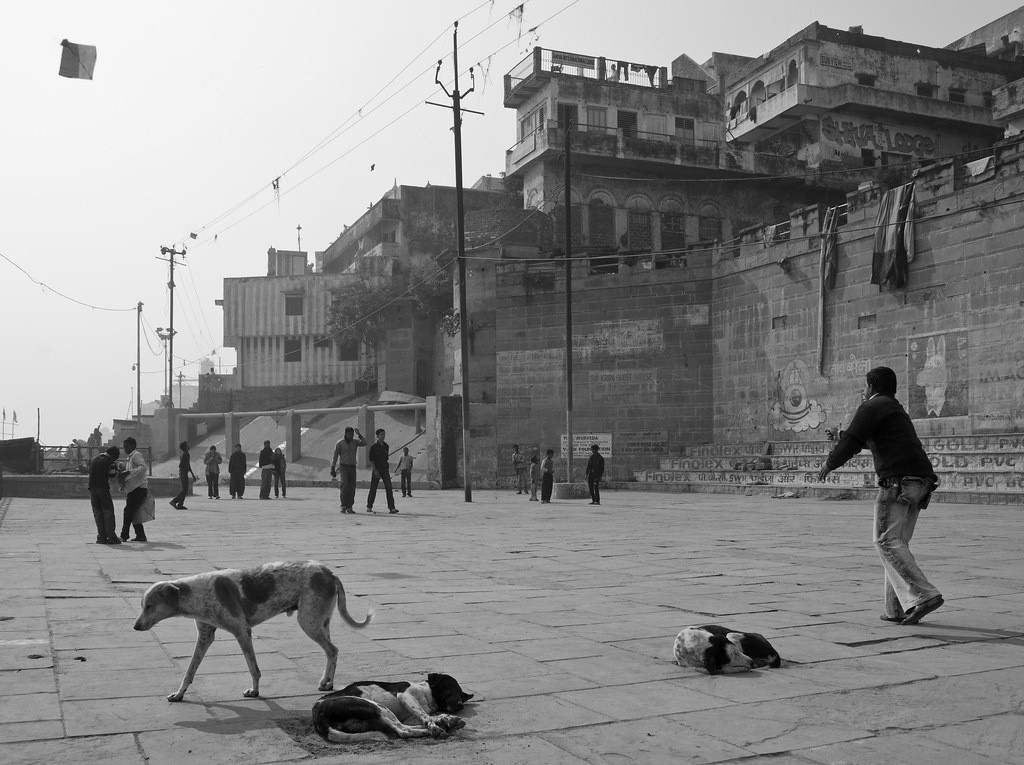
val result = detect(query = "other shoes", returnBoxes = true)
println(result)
[901,595,944,625]
[131,536,147,541]
[259,493,288,499]
[367,507,372,512]
[402,494,406,497]
[177,504,188,509]
[237,496,242,499]
[340,506,346,513]
[588,501,601,506]
[169,500,178,510]
[529,498,540,501]
[208,496,212,499]
[541,500,551,504]
[880,614,907,622]
[389,509,399,514]
[119,537,126,542]
[96,538,109,544]
[524,490,529,494]
[232,495,236,498]
[216,496,220,499]
[516,491,522,494]
[347,507,355,514]
[407,493,412,497]
[107,538,121,544]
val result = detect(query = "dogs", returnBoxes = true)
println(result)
[134,560,374,702]
[674,625,781,674]
[117,462,130,491]
[312,673,475,743]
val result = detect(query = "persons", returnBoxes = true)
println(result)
[272,448,286,498]
[512,445,529,494]
[228,444,246,499]
[169,441,196,510]
[585,445,605,505]
[89,446,122,544]
[540,449,555,504]
[530,456,539,501]
[330,427,367,514]
[367,429,399,514]
[819,366,944,625]
[119,438,148,542]
[394,447,413,497]
[259,440,274,500]
[204,446,222,499]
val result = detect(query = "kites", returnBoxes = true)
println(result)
[59,39,97,80]
